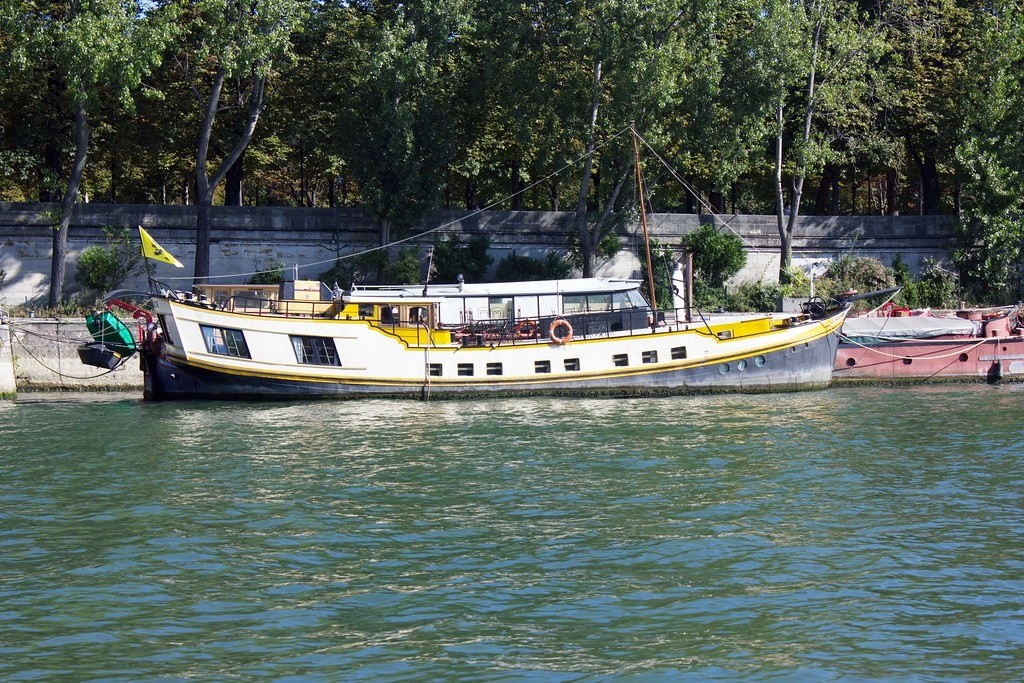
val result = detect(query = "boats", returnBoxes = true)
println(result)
[697,287,1024,385]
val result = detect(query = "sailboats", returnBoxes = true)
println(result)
[138,121,850,400]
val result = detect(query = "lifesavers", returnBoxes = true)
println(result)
[882,302,899,318]
[516,319,538,340]
[549,317,573,344]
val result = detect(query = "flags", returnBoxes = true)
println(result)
[139,226,185,268]
